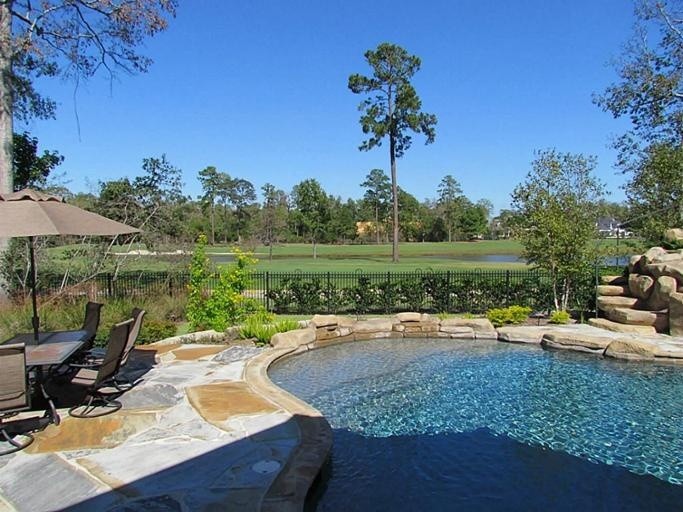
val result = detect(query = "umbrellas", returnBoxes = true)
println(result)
[0,185,144,345]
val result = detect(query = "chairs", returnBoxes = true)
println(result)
[0,301,147,455]
